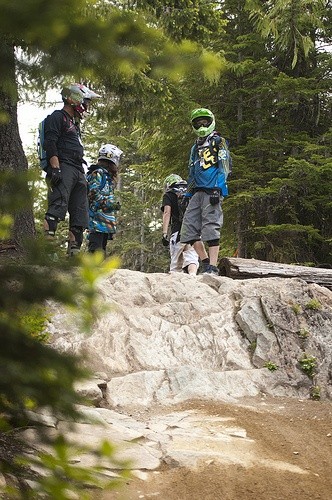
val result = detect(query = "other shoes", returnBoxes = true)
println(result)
[197,257,219,276]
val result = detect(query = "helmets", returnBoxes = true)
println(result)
[60,82,102,119]
[164,174,188,190]
[97,144,123,167]
[190,108,215,137]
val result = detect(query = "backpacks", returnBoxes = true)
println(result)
[38,109,70,172]
[166,187,189,223]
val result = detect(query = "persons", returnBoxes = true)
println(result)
[42,83,103,258]
[86,143,124,262]
[160,173,199,277]
[178,107,231,277]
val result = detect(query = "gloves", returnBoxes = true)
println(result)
[50,168,63,185]
[210,189,219,206]
[161,233,169,246]
[181,195,189,206]
